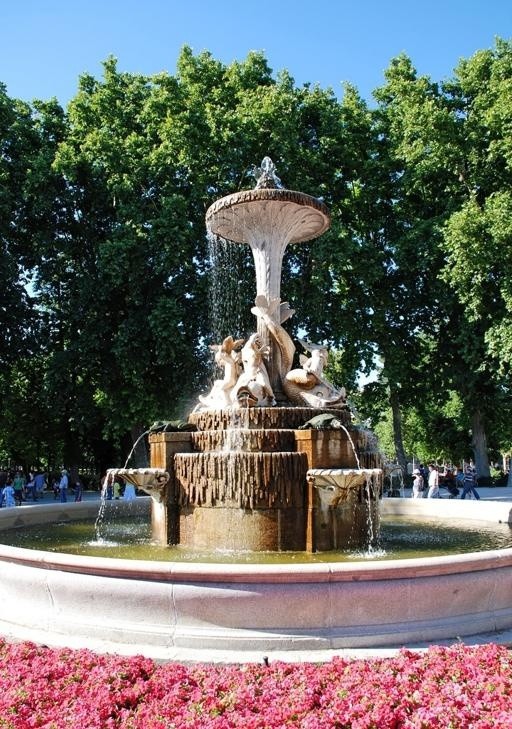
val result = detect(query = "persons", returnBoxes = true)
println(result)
[0,468,136,508]
[412,465,480,500]
